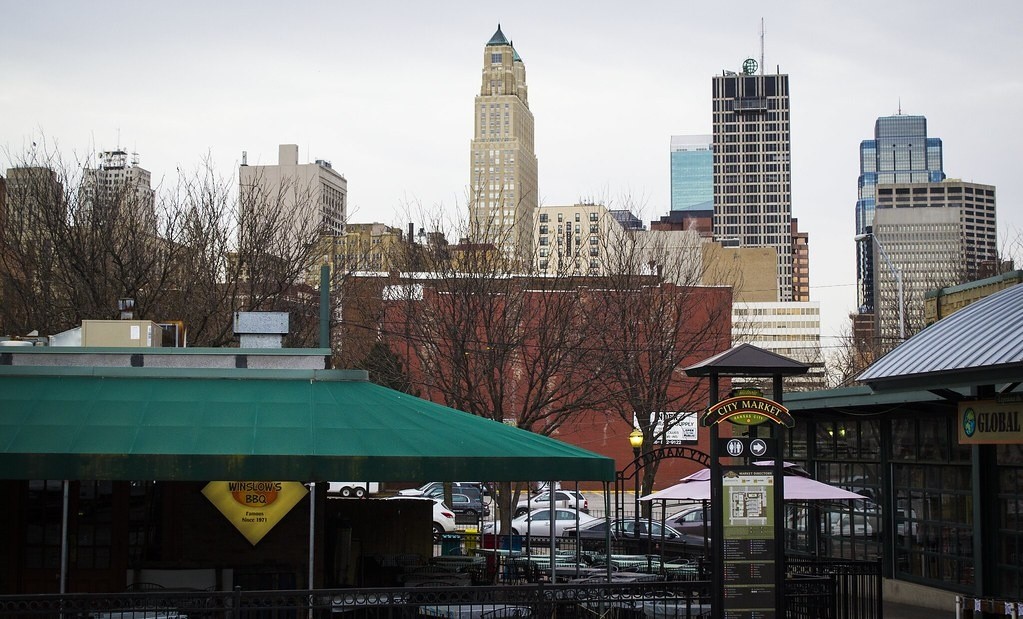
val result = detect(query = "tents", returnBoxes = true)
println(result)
[0,365,617,619]
[636,460,870,579]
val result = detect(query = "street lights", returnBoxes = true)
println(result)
[855,233,906,344]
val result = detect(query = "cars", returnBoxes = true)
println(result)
[328,480,712,560]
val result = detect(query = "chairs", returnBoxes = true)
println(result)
[627,522,647,533]
[113,547,712,619]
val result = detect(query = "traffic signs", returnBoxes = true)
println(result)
[750,439,767,456]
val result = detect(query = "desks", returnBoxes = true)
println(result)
[545,567,608,576]
[568,578,636,583]
[509,558,565,562]
[636,565,711,575]
[521,583,599,598]
[404,579,470,587]
[610,560,660,568]
[434,554,486,563]
[78,611,188,619]
[582,595,683,619]
[596,572,657,581]
[536,563,586,570]
[521,555,577,557]
[413,573,469,579]
[404,563,461,573]
[644,604,791,619]
[418,605,530,619]
[470,548,521,584]
[316,595,408,619]
[605,555,661,561]
